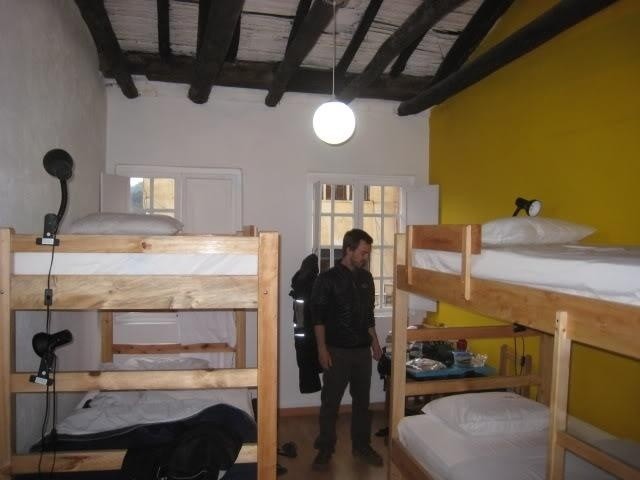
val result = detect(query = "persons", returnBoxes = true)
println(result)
[308,228,387,470]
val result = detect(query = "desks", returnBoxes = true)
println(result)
[381,349,499,428]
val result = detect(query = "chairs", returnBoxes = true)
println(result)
[500,345,532,396]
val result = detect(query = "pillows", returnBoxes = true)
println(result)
[70,212,183,234]
[422,389,550,437]
[482,219,598,245]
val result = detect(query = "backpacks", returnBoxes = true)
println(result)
[289,254,321,395]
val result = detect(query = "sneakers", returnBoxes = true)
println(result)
[313,441,384,468]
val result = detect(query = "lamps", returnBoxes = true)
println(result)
[38,148,73,245]
[313,0,357,146]
[513,197,540,217]
[29,330,71,392]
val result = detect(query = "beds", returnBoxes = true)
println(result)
[388,227,640,480]
[0,224,280,480]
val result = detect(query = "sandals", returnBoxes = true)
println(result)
[278,439,297,475]
